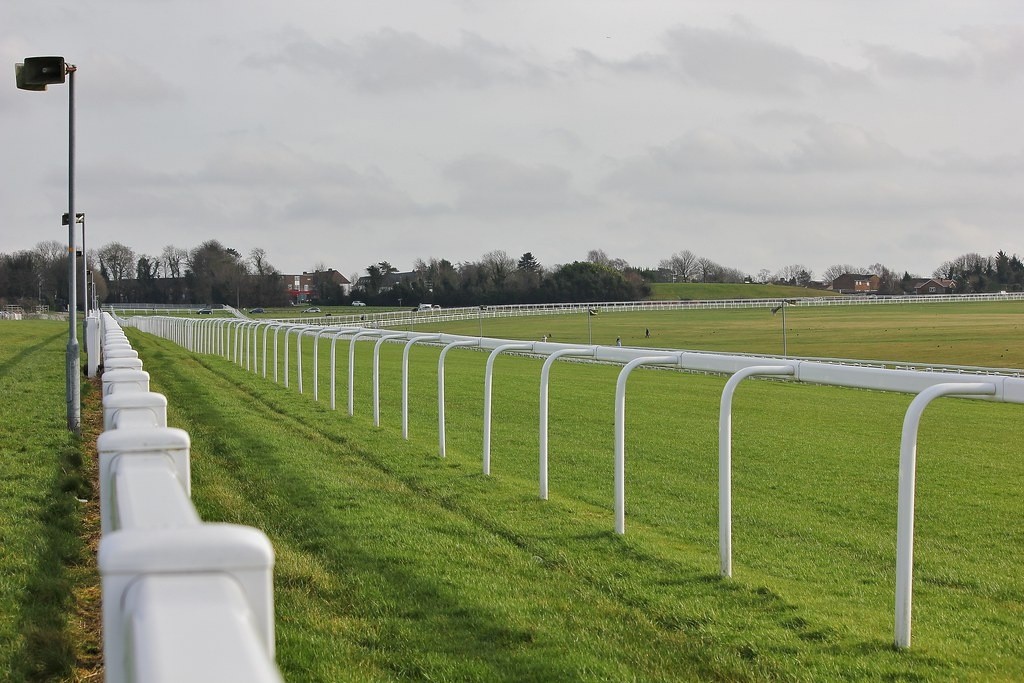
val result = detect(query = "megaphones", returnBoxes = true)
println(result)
[87,270,90,275]
[62,214,82,224]
[16,58,65,91]
[76,251,82,257]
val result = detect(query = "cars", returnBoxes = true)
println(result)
[196,308,212,314]
[304,307,321,312]
[352,301,366,306]
[249,308,264,313]
[418,304,441,311]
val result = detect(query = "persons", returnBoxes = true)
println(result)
[542,335,547,342]
[616,336,621,346]
[645,328,650,338]
